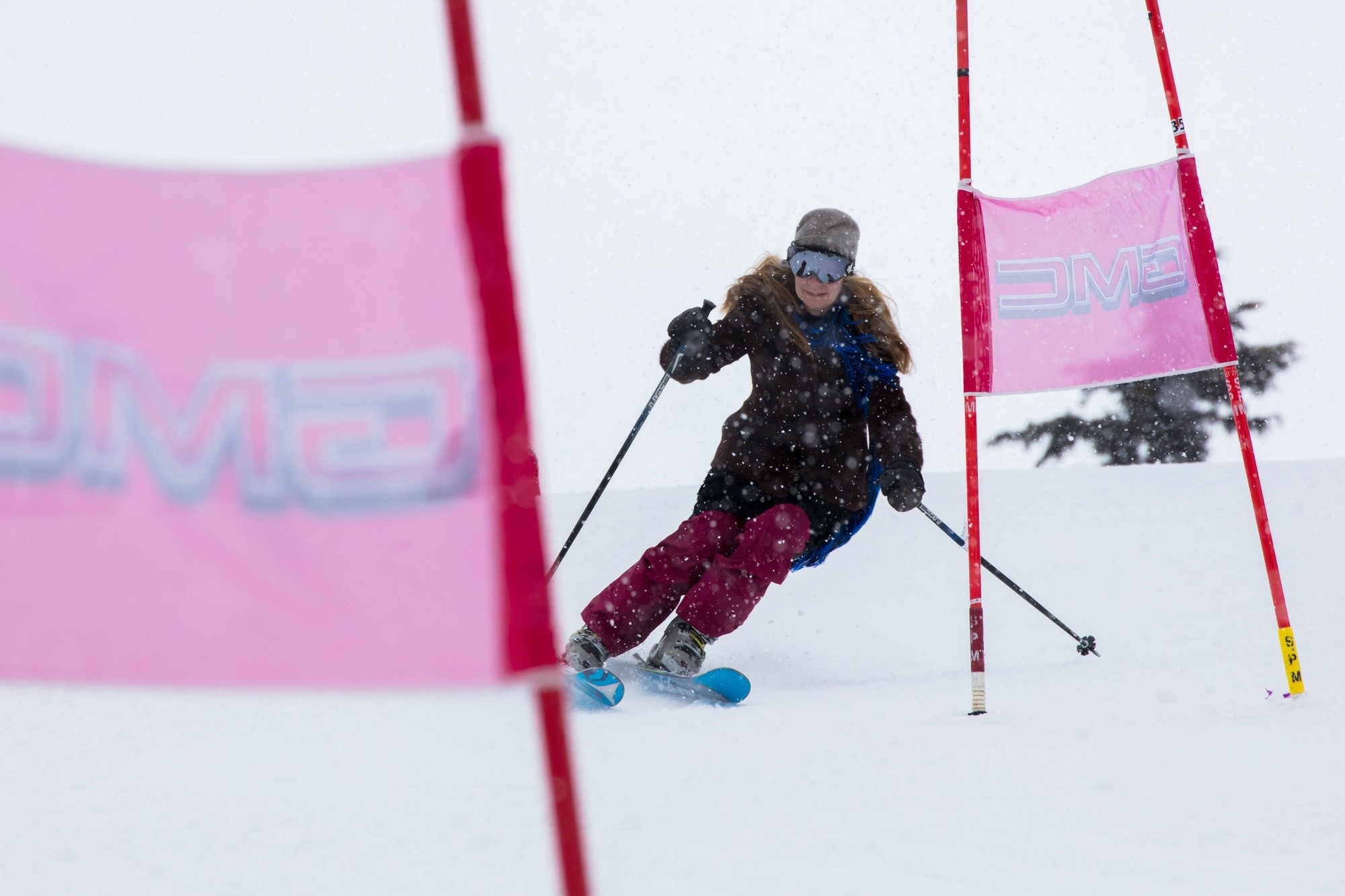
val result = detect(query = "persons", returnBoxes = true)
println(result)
[565,208,924,677]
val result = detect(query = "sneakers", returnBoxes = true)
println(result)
[567,625,607,670]
[649,617,716,674]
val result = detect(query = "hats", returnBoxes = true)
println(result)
[795,208,859,261]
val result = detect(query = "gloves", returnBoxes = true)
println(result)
[880,468,926,512]
[668,307,712,356]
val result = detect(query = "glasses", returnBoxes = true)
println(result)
[788,246,851,282]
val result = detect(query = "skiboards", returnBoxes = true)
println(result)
[562,661,755,711]
